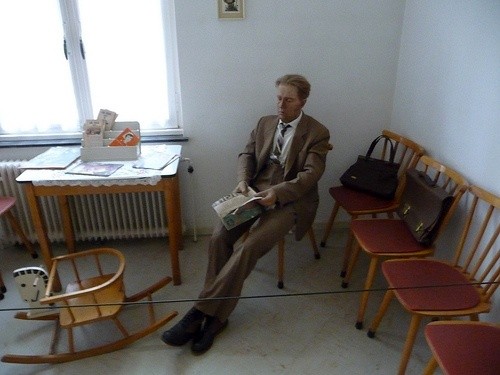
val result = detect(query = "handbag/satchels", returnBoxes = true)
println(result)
[339,134,401,201]
[396,175,454,248]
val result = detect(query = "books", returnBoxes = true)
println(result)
[211,186,263,230]
[97,109,118,131]
[108,128,140,146]
[84,119,105,147]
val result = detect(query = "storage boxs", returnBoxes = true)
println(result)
[80,121,142,161]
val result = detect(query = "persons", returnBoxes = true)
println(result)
[161,74,329,354]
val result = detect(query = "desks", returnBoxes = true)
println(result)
[15,145,183,286]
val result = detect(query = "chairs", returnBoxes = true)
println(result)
[0,248,178,364]
[370,184,500,375]
[342,155,470,330]
[425,320,500,375]
[321,130,425,278]
[0,196,38,293]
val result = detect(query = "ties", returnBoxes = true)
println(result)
[274,123,292,161]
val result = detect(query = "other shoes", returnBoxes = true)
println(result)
[161,307,205,346]
[191,319,228,353]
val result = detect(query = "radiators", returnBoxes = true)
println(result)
[0,160,169,244]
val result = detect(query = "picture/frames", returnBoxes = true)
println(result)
[217,0,246,20]
